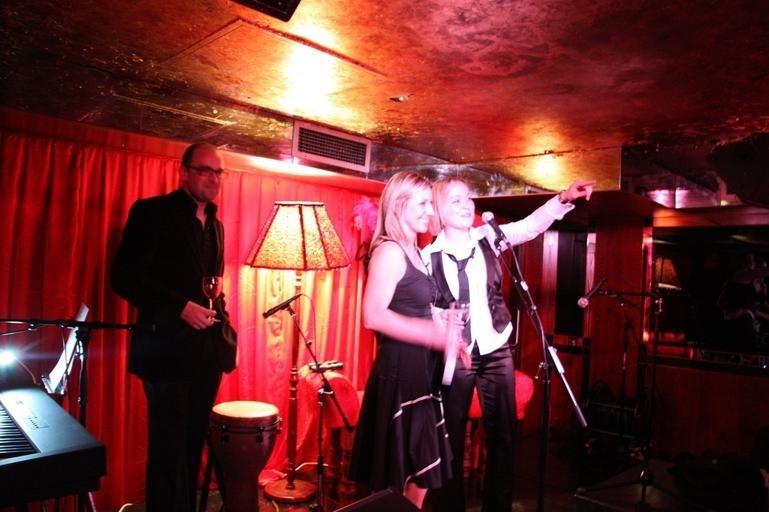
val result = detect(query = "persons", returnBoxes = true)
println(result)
[421,175,598,511]
[107,142,227,512]
[346,171,472,511]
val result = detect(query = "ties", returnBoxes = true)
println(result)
[447,247,476,346]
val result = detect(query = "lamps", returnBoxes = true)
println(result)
[245,201,353,504]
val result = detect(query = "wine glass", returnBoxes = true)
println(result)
[201,275,223,324]
[448,301,472,346]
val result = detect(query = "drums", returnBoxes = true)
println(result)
[209,401,281,511]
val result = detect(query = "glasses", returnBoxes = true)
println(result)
[184,162,229,181]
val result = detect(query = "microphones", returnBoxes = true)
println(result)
[482,210,510,246]
[263,294,302,318]
[577,272,609,309]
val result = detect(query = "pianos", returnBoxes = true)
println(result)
[0,388,106,497]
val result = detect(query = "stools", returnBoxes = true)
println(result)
[446,367,534,512]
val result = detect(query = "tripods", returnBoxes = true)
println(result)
[575,283,713,512]
